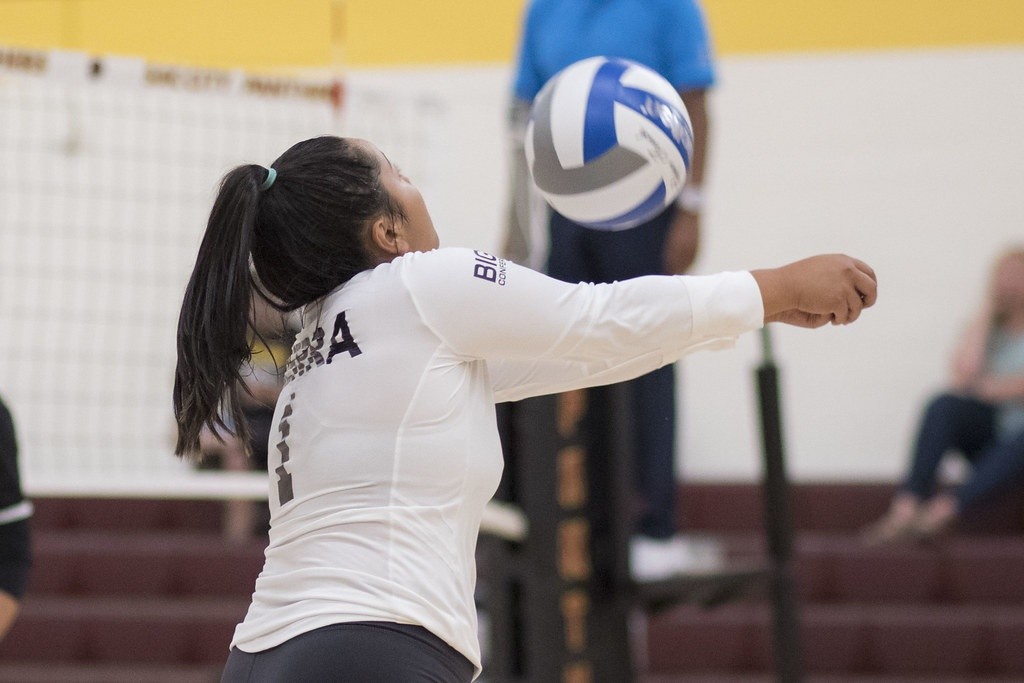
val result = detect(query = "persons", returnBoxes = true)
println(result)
[0,399,35,640]
[506,0,717,585]
[173,135,877,683]
[864,246,1024,544]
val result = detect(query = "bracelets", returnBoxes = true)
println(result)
[678,186,705,215]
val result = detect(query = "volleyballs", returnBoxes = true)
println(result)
[521,52,699,236]
